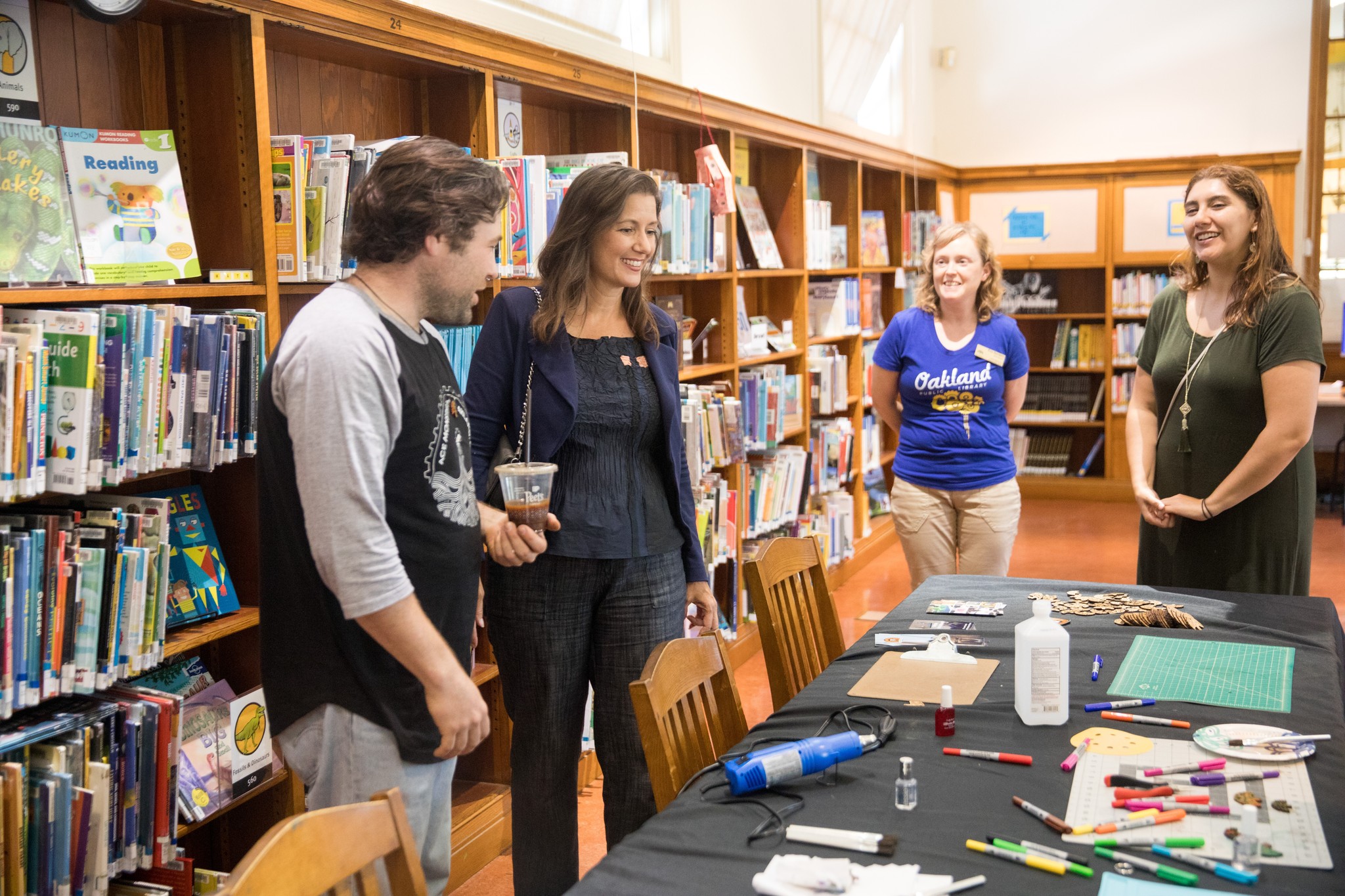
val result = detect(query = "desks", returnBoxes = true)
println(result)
[562,575,1345,896]
[1311,382,1345,510]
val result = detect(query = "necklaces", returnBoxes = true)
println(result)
[1177,276,1235,453]
[351,273,428,342]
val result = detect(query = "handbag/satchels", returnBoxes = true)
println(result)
[485,286,543,510]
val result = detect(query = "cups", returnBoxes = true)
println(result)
[493,461,559,534]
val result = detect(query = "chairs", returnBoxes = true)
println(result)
[628,625,750,814]
[213,786,430,896]
[741,532,848,712]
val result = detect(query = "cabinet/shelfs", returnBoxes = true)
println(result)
[0,0,1296,896]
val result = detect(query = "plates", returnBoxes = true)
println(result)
[1192,723,1316,762]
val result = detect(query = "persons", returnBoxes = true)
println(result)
[871,221,1031,593]
[467,162,719,895]
[263,139,561,895]
[1126,166,1328,598]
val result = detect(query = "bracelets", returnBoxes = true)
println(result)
[1202,498,1214,520]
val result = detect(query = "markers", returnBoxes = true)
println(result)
[1190,771,1279,786]
[1012,796,1072,834]
[1094,839,1203,847]
[966,839,1066,875]
[943,748,1032,765]
[921,875,986,896]
[786,824,898,856]
[1101,711,1190,728]
[1144,758,1226,777]
[1152,845,1258,883]
[1092,655,1102,681]
[1061,738,1090,770]
[1084,699,1155,712]
[1020,840,1088,866]
[1094,846,1198,884]
[987,836,1093,877]
[1125,800,1229,812]
[1072,808,1185,834]
[1112,795,1209,807]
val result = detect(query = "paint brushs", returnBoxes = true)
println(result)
[1229,734,1331,746]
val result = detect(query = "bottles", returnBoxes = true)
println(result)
[1013,599,1070,727]
[934,685,955,736]
[894,756,917,811]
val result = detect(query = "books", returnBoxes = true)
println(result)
[1007,374,1105,477]
[642,169,726,275]
[684,557,738,640]
[269,133,471,282]
[1112,269,1179,413]
[497,150,628,278]
[0,122,203,286]
[0,302,266,502]
[739,363,802,623]
[1052,319,1105,369]
[431,322,482,395]
[690,472,737,568]
[862,210,891,484]
[803,198,861,569]
[0,482,244,720]
[0,656,284,896]
[679,380,746,487]
[1002,267,1059,314]
